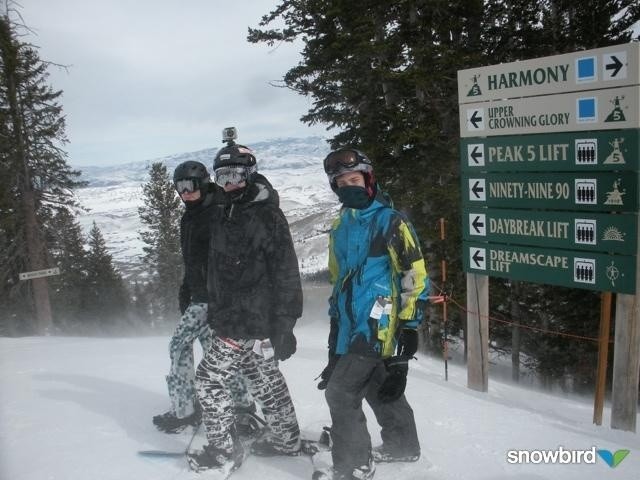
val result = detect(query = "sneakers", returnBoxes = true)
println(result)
[372,445,420,463]
[333,454,374,480]
[191,444,230,470]
[153,402,200,430]
[250,437,276,455]
[236,424,254,435]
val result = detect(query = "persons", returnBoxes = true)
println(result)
[187,142,305,480]
[152,160,259,441]
[309,145,432,480]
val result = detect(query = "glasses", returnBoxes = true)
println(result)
[213,163,257,187]
[175,178,204,194]
[323,151,373,174]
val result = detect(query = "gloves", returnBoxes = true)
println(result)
[398,330,418,358]
[318,358,341,390]
[376,354,412,405]
[273,315,297,362]
[179,285,190,313]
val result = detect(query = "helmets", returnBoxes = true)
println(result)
[328,149,373,183]
[173,162,210,207]
[211,144,255,168]
